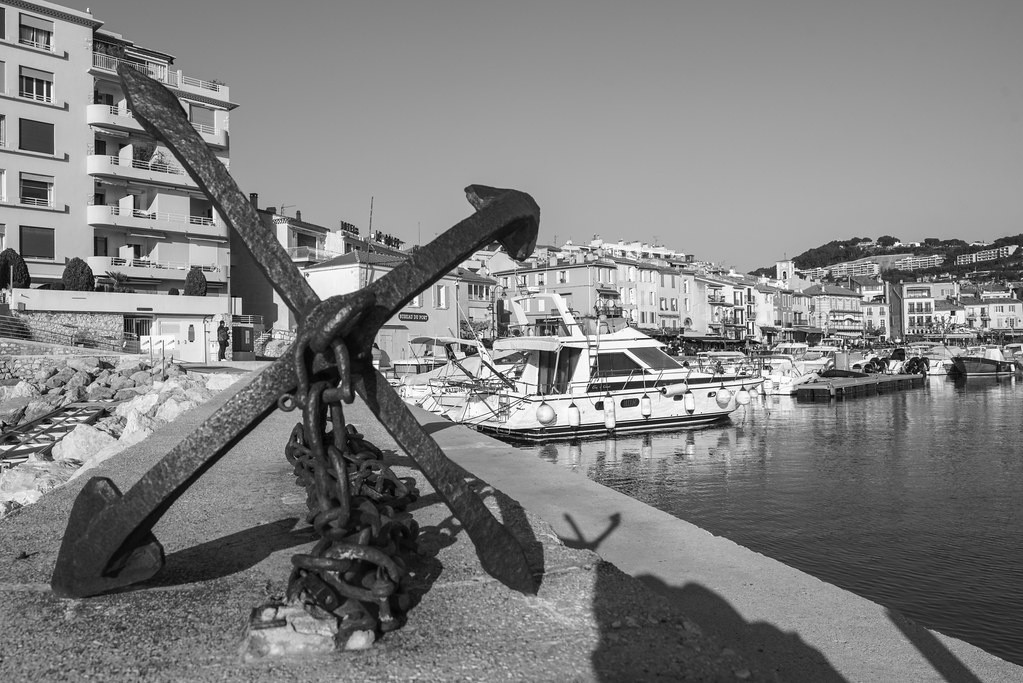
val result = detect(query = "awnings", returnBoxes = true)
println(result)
[797,329,823,333]
[760,327,777,332]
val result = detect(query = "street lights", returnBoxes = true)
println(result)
[478,285,507,341]
[592,299,617,315]
[715,306,733,351]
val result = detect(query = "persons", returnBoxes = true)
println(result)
[217,321,230,361]
[713,361,724,374]
[683,361,689,367]
[668,340,697,356]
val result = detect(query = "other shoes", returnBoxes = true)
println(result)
[222,358,226,361]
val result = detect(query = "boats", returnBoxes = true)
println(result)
[888,342,953,375]
[386,293,843,441]
[951,344,1018,377]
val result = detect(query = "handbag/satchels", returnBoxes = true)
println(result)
[225,341,229,347]
